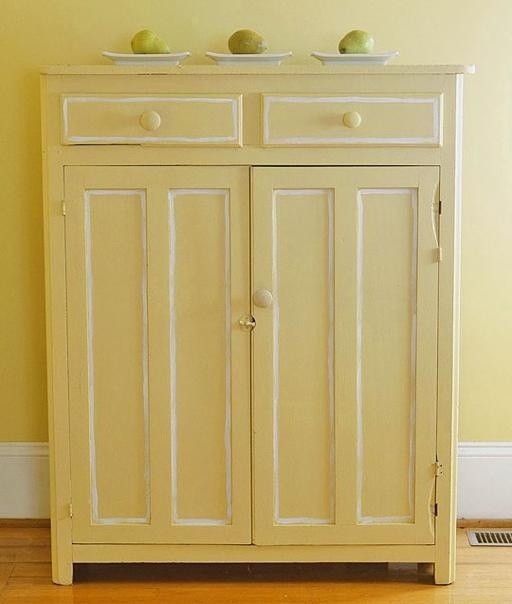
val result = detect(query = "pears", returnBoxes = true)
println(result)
[229,30,268,54]
[338,30,376,54]
[131,29,172,54]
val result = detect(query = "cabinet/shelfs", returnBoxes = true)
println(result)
[38,65,474,585]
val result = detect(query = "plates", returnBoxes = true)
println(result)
[205,50,293,66]
[102,49,191,65]
[310,50,400,66]
